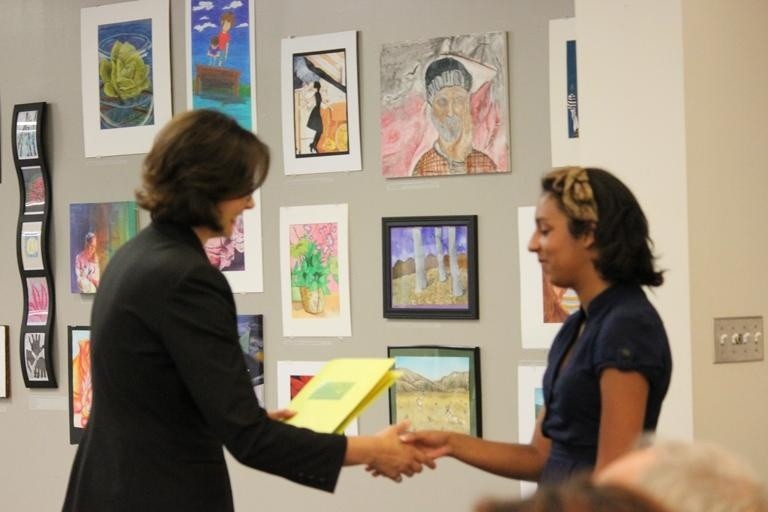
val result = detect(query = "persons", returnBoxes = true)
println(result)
[595,437,766,511]
[305,81,323,153]
[73,234,101,292]
[216,11,234,69]
[408,58,501,178]
[60,109,436,511]
[465,477,668,511]
[208,36,220,67]
[365,167,671,486]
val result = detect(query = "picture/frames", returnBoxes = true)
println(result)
[386,341,484,440]
[7,98,59,391]
[378,213,482,324]
[67,321,98,446]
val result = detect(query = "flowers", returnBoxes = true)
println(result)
[100,39,152,103]
[290,236,339,295]
